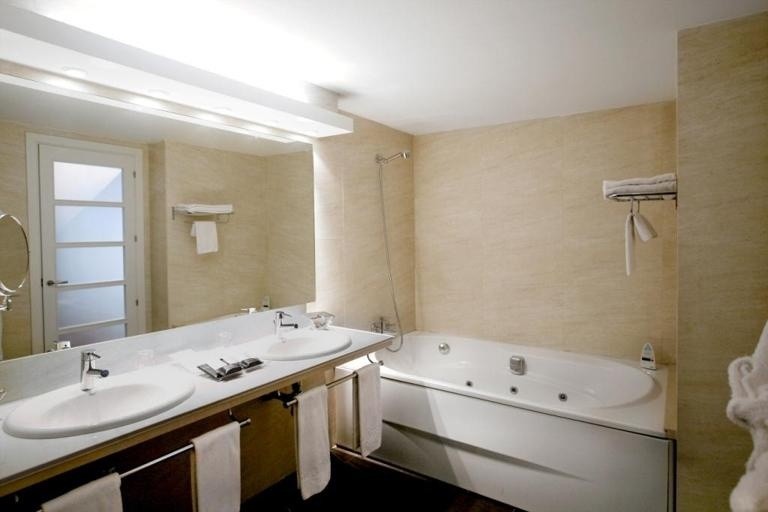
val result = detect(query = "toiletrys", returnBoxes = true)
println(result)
[640,341,657,372]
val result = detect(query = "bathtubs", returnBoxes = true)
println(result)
[369,328,659,415]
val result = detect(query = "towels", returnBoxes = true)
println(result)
[175,201,233,256]
[350,363,383,456]
[40,473,125,512]
[602,172,678,278]
[294,385,332,501]
[723,318,767,512]
[190,422,240,512]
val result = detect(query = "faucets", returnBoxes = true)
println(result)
[79,348,108,391]
[240,307,258,314]
[372,317,398,333]
[272,309,297,336]
[48,340,71,349]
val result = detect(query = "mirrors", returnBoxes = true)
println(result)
[0,59,319,366]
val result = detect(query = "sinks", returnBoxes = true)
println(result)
[260,330,351,362]
[3,364,195,438]
